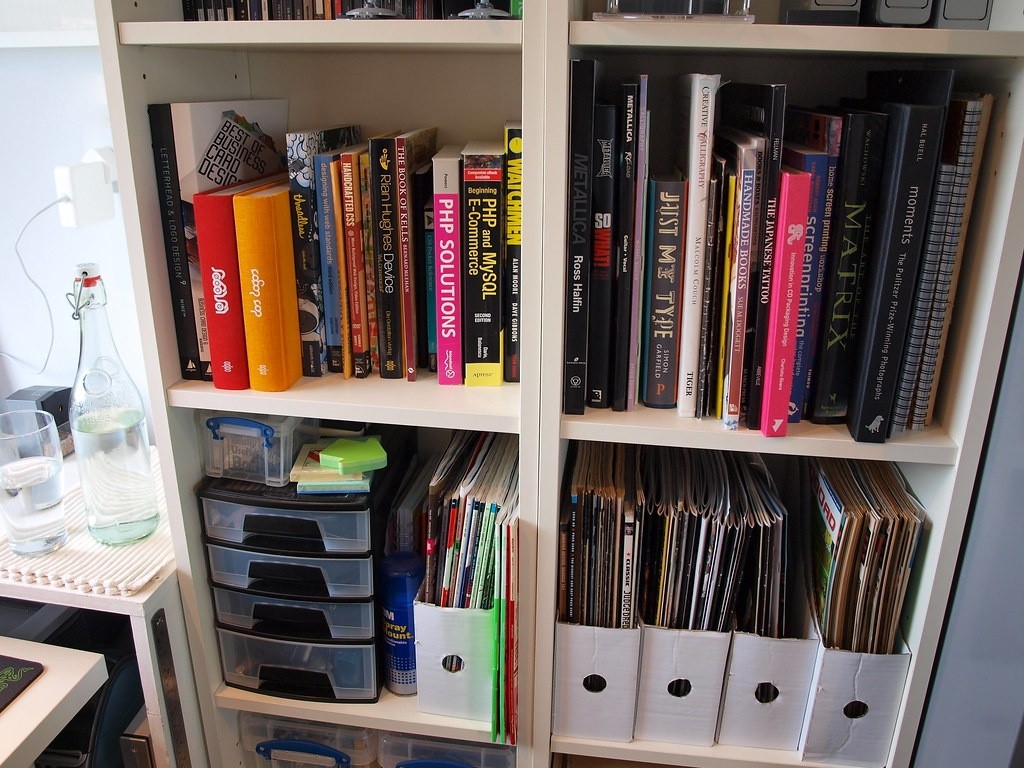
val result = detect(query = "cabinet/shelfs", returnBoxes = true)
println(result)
[92,1,1023,768]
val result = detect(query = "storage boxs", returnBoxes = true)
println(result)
[377,729,516,768]
[239,710,378,768]
[550,455,911,765]
[413,573,494,723]
[197,424,418,705]
[198,408,320,487]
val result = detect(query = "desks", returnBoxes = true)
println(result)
[0,636,110,768]
[1,441,210,768]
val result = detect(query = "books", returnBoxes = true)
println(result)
[146,0,997,754]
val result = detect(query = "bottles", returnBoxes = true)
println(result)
[69,261,157,545]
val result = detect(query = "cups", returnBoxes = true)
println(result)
[0,409,69,556]
